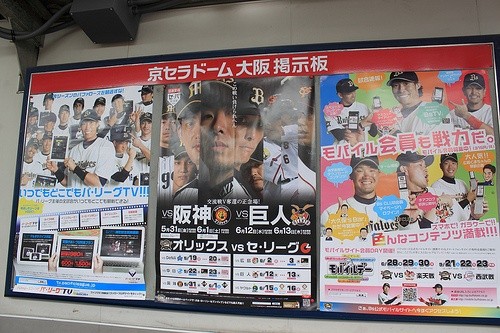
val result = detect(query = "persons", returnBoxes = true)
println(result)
[320,151,488,235]
[442,73,494,137]
[387,71,434,135]
[21,85,154,188]
[378,281,449,307]
[158,79,314,206]
[325,79,377,145]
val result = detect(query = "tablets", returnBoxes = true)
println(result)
[17,231,56,265]
[56,236,99,273]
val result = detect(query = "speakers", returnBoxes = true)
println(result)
[69,0,140,45]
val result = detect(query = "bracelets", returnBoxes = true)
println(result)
[467,116,483,130]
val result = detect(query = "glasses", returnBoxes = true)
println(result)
[290,108,314,119]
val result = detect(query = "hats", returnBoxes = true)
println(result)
[140,112,152,122]
[42,131,53,140]
[161,103,177,117]
[59,105,70,114]
[27,107,38,117]
[440,153,458,163]
[236,77,314,117]
[386,72,419,86]
[350,153,380,169]
[397,152,435,166]
[432,284,442,288]
[175,146,188,159]
[249,150,264,164]
[202,77,233,92]
[115,132,131,143]
[80,109,99,125]
[27,138,40,148]
[42,93,54,105]
[335,79,359,94]
[93,98,105,108]
[383,283,390,287]
[72,98,84,109]
[138,86,154,95]
[42,113,57,123]
[111,94,122,103]
[175,81,203,125]
[463,73,485,89]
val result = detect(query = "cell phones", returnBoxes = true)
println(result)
[469,170,477,187]
[397,171,410,210]
[349,110,359,134]
[435,87,444,104]
[373,96,381,111]
[474,182,485,214]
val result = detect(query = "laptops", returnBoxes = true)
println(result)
[96,226,146,274]
